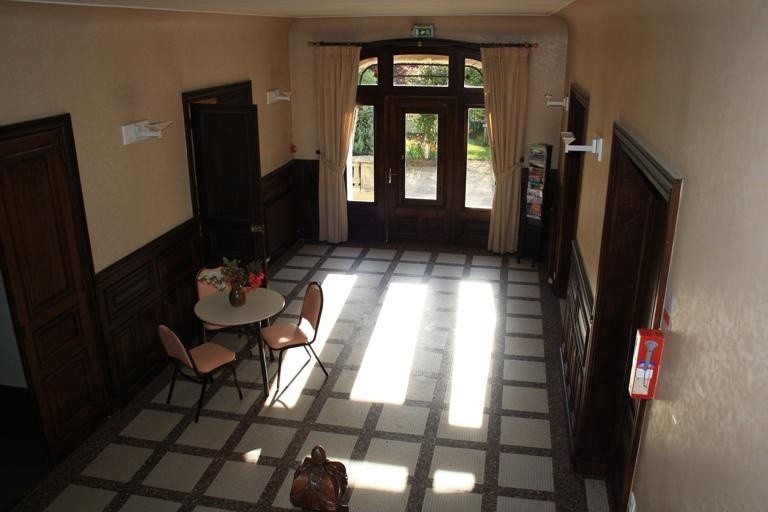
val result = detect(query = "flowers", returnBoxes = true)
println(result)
[221,256,248,288]
[198,263,265,291]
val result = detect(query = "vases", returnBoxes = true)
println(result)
[228,284,246,306]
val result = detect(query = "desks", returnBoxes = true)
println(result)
[194,287,286,397]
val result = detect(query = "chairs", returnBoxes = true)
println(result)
[195,265,251,363]
[159,324,244,422]
[260,282,328,390]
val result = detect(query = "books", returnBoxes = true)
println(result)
[524,143,548,221]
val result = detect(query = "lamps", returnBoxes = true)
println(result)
[560,131,603,162]
[122,120,173,146]
[545,94,569,111]
[266,88,293,104]
